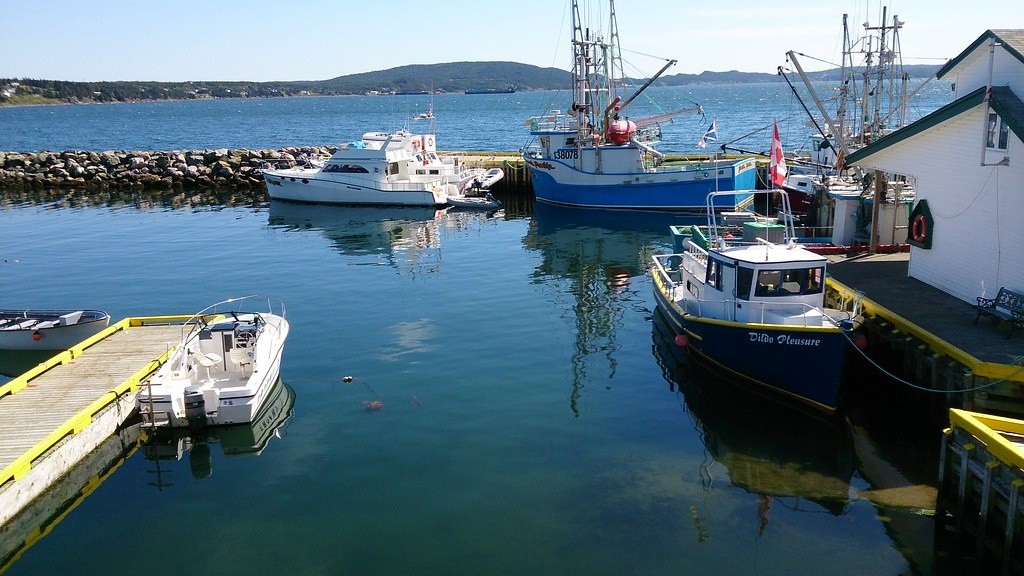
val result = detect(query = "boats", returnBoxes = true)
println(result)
[646,188,866,419]
[0,307,110,351]
[649,308,863,518]
[447,190,501,209]
[257,113,465,207]
[137,294,290,430]
[414,81,436,120]
[0,351,65,378]
[464,84,518,94]
[523,1,757,213]
[464,166,505,189]
[138,379,297,461]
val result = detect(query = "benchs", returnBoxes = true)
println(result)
[974,286,1024,339]
[32,319,61,329]
[0,320,8,326]
[4,319,38,330]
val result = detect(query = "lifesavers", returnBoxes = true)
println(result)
[911,216,925,240]
[413,139,420,149]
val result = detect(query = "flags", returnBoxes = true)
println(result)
[701,123,717,145]
[770,123,787,188]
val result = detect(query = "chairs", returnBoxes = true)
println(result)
[229,349,254,382]
[194,350,223,382]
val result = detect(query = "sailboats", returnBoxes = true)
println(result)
[718,0,910,226]
